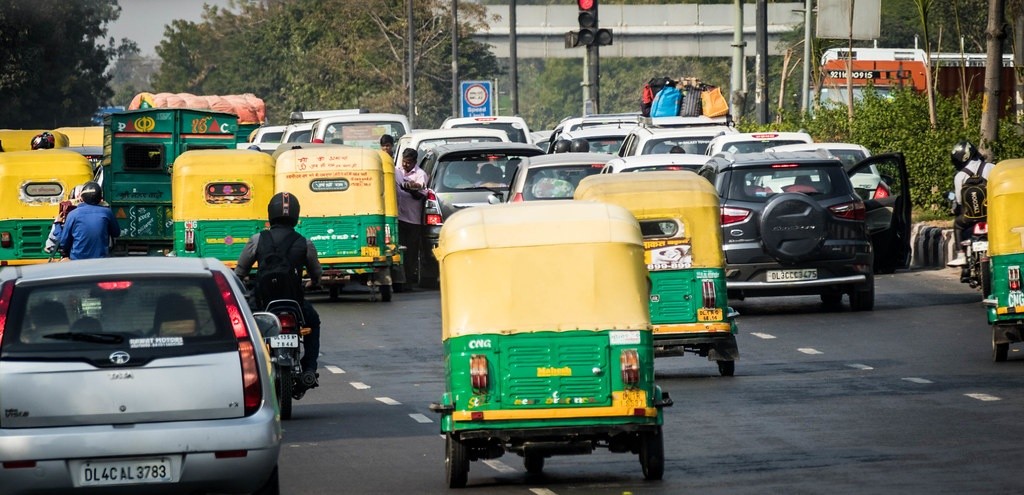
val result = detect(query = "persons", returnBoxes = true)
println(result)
[570,138,590,153]
[45,185,85,252]
[31,134,48,150]
[381,134,394,157]
[42,131,54,148]
[393,148,428,292]
[947,141,995,267]
[233,192,322,378]
[556,140,572,154]
[54,181,120,261]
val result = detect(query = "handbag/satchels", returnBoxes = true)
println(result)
[639,77,729,117]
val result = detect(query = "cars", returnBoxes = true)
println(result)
[236,109,914,316]
[0,256,282,495]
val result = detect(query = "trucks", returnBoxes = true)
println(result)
[101,110,239,258]
[803,48,932,118]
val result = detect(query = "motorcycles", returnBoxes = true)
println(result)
[431,200,667,491]
[986,157,1024,362]
[573,170,741,377]
[0,107,407,303]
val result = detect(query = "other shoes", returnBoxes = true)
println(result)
[947,256,967,267]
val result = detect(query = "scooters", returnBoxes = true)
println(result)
[960,219,990,307]
[260,300,316,423]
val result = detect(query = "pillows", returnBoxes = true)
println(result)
[781,185,818,192]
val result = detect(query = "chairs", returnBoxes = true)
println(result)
[25,301,72,342]
[533,177,575,197]
[145,293,200,338]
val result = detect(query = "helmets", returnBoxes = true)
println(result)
[31,135,48,149]
[82,181,101,205]
[68,185,83,199]
[43,132,55,147]
[267,192,299,227]
[951,139,980,170]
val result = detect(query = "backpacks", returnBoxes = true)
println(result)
[960,161,988,224]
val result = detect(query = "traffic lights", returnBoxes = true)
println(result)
[565,0,613,49]
